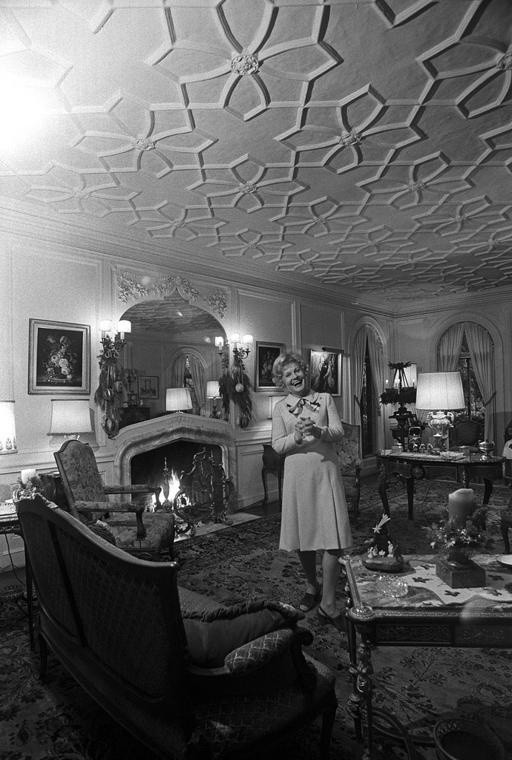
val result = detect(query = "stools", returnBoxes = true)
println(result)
[261,445,285,512]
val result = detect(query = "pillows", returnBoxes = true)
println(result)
[182,601,305,668]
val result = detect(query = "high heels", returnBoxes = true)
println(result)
[317,606,347,634]
[301,589,320,610]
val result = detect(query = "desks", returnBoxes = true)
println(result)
[378,451,507,525]
[0,501,28,586]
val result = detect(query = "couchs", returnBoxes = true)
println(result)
[17,493,338,758]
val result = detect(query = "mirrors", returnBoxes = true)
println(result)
[109,286,230,432]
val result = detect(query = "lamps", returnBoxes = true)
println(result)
[392,362,417,430]
[47,399,94,441]
[0,399,18,456]
[416,372,466,455]
[207,381,221,418]
[117,319,131,342]
[165,387,194,411]
[231,334,254,359]
[214,336,225,359]
[98,319,116,343]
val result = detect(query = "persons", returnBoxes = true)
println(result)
[270,351,356,632]
[311,353,338,394]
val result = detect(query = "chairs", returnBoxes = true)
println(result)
[54,440,175,559]
[336,422,363,516]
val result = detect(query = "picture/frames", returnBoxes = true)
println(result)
[137,376,159,400]
[307,347,344,398]
[255,341,286,393]
[28,318,92,395]
